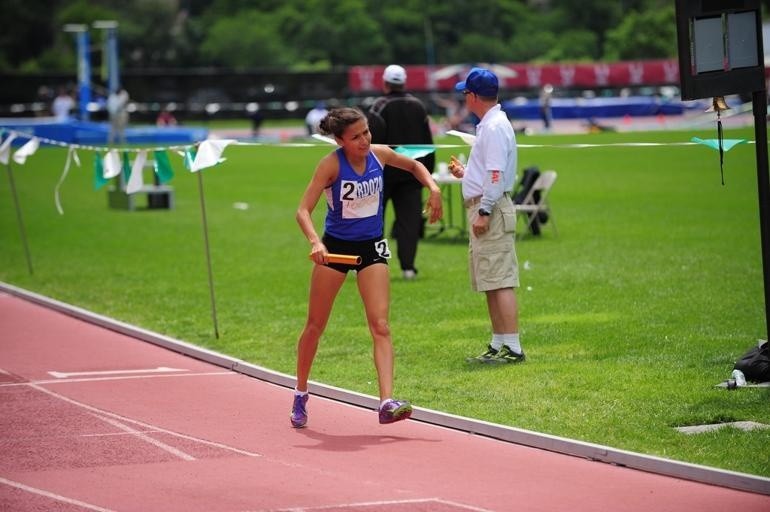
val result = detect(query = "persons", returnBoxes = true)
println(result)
[365,64,436,279]
[51,87,77,120]
[289,107,443,427]
[107,85,130,146]
[447,66,525,363]
[437,62,491,137]
[154,104,179,126]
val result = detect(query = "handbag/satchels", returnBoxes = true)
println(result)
[732,339,770,384]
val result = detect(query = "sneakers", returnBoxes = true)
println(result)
[466,343,499,362]
[480,343,525,364]
[404,268,416,280]
[289,386,309,428]
[378,398,412,424]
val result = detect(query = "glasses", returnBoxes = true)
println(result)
[461,90,471,95]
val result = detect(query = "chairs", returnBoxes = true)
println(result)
[513,171,558,241]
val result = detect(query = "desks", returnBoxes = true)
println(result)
[422,173,470,243]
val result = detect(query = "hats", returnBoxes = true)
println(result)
[454,66,499,96]
[382,64,408,85]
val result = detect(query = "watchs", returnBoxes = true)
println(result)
[478,208,490,217]
